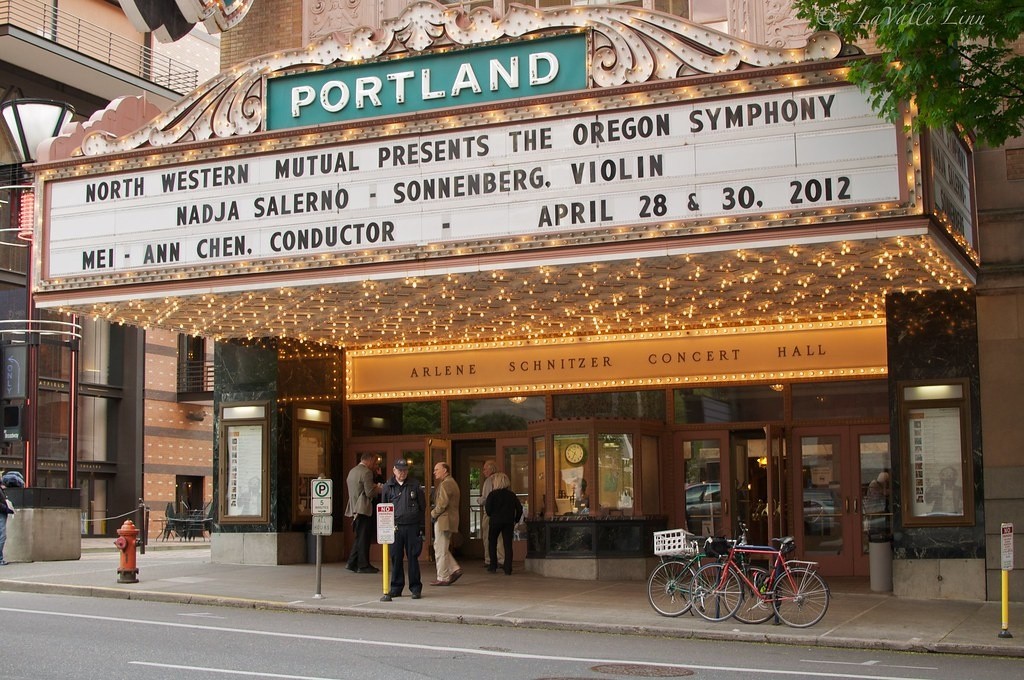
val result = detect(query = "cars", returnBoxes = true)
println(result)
[797,490,889,537]
[685,482,723,525]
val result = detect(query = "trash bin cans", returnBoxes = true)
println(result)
[867,525,891,594]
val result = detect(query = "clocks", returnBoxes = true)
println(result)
[566,443,587,465]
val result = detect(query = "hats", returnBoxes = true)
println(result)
[394,459,409,470]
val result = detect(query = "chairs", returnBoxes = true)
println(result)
[164,505,214,542]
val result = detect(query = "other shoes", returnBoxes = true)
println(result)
[498,564,503,567]
[412,592,421,598]
[430,580,449,586]
[448,568,464,583]
[504,570,512,575]
[387,590,401,597]
[359,565,379,573]
[487,568,496,573]
[480,564,489,567]
[345,563,358,572]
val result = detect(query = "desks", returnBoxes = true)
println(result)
[153,519,173,541]
[176,517,213,541]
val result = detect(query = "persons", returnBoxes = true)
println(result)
[344,451,383,573]
[477,459,505,569]
[382,459,425,599]
[863,472,890,553]
[486,472,523,575]
[428,462,463,586]
[0,476,14,565]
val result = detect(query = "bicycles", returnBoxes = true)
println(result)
[688,527,834,628]
[647,515,776,624]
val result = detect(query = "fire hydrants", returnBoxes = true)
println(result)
[113,518,143,584]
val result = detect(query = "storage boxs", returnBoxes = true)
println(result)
[653,529,695,556]
